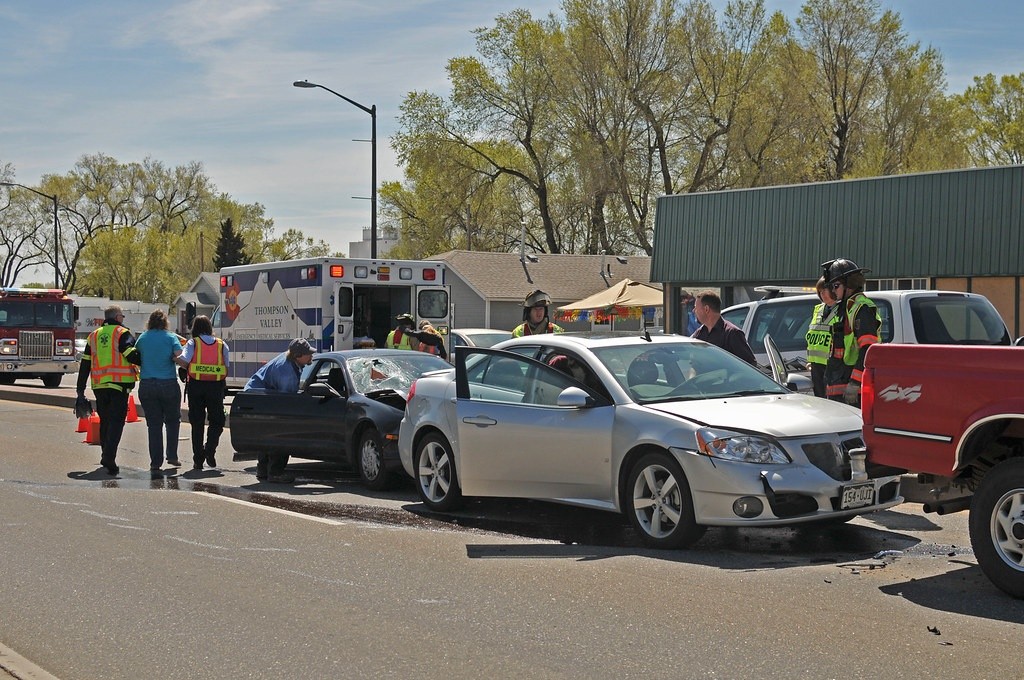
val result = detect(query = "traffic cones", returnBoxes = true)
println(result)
[82,408,99,443]
[125,396,141,422]
[74,416,89,432]
[88,416,102,445]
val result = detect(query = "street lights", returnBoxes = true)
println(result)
[0,182,58,289]
[293,79,377,259]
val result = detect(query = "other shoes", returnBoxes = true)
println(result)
[167,460,181,466]
[194,463,203,468]
[205,449,216,467]
[151,465,158,470]
[100,460,119,471]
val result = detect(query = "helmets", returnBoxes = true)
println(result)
[820,257,871,287]
[395,313,414,330]
[524,288,552,321]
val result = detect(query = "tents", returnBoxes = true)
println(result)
[554,277,663,332]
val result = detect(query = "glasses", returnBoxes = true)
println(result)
[119,315,125,318]
[834,282,843,288]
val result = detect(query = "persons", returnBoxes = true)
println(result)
[805,275,839,399]
[820,258,883,409]
[417,320,444,356]
[76,303,141,471]
[243,338,318,484]
[384,312,419,351]
[511,289,565,338]
[172,314,229,469]
[134,308,189,470]
[688,290,757,379]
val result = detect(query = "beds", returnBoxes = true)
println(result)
[354,336,375,350]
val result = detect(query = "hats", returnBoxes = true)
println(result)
[289,338,317,355]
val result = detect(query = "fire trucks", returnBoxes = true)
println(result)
[0,287,82,387]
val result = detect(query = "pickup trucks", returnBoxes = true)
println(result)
[859,343,1024,600]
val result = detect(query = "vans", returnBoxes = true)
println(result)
[655,286,1014,400]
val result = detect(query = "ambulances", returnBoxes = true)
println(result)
[186,257,456,388]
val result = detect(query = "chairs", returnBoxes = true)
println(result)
[628,359,669,388]
[771,321,790,342]
[538,356,589,400]
[488,359,524,389]
[328,368,344,393]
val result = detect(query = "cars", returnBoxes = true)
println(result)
[397,331,904,548]
[229,349,456,491]
[448,328,550,379]
[75,339,88,362]
